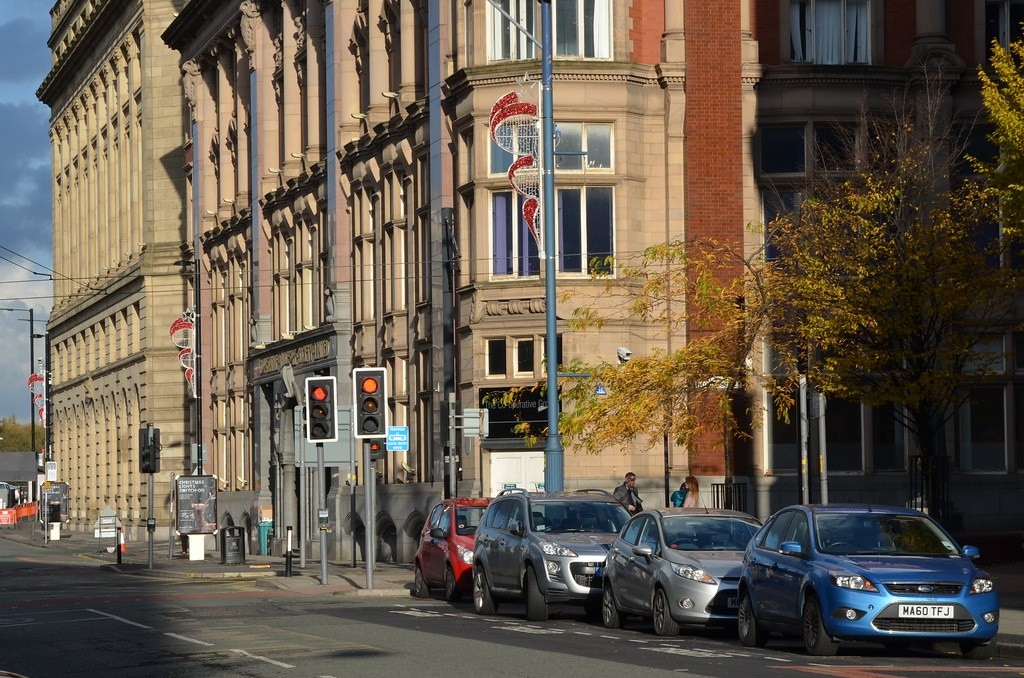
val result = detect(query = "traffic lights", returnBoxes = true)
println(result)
[305,376,338,443]
[352,368,388,439]
[371,442,385,459]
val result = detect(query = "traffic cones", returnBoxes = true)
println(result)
[120,525,126,552]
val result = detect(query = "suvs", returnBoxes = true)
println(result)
[472,487,631,622]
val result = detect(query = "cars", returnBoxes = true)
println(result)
[414,496,494,601]
[602,506,764,636]
[736,502,998,661]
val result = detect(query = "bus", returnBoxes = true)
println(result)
[0,482,15,511]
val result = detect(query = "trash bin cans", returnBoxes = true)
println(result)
[257,520,275,556]
[220,526,246,565]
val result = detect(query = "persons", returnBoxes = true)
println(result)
[613,472,643,515]
[180,535,188,555]
[670,476,700,507]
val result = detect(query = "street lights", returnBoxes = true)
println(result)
[30,333,43,451]
[174,258,204,479]
